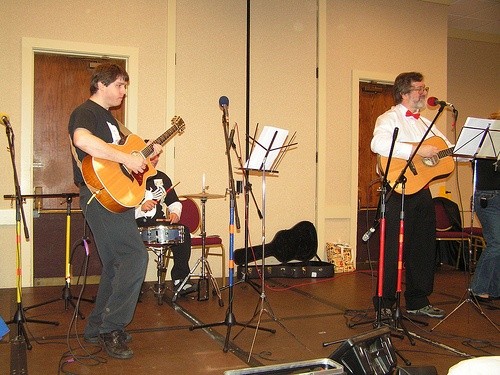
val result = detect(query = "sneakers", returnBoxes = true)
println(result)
[409,304,446,318]
[376,308,392,320]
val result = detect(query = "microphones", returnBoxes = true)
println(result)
[0,110,11,128]
[362,220,380,241]
[218,96,229,123]
[225,129,235,155]
[427,97,454,107]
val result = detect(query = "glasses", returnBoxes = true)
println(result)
[405,86,429,93]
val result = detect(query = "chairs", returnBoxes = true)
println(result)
[462,194,486,266]
[432,196,470,272]
[161,195,225,288]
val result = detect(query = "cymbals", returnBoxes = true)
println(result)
[177,192,225,199]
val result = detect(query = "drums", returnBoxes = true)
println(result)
[137,225,185,245]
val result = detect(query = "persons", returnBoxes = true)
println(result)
[370,72,473,318]
[67,63,163,359]
[135,139,195,295]
[469,112,500,302]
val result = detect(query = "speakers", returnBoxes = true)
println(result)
[328,326,398,375]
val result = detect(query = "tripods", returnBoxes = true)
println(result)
[188,112,276,353]
[1,127,59,351]
[168,200,223,307]
[5,194,95,318]
[320,106,500,366]
[214,131,300,296]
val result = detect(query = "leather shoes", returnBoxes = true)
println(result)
[491,294,500,299]
[88,330,132,342]
[98,331,132,358]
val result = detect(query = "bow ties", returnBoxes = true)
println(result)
[405,110,420,119]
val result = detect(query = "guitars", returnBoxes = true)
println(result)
[80,115,186,214]
[378,136,457,195]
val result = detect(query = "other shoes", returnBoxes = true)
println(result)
[470,291,494,303]
[173,279,196,294]
